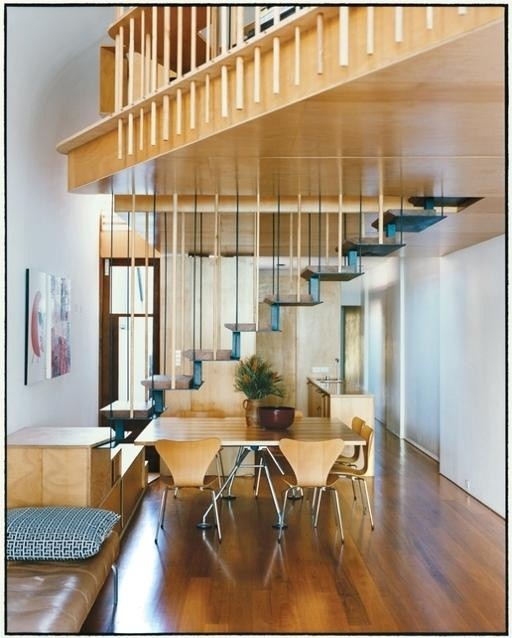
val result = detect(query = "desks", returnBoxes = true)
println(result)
[134,417,368,529]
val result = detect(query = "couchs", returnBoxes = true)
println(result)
[6,529,121,635]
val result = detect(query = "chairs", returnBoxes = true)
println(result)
[310,424,376,531]
[273,437,348,543]
[257,410,305,499]
[171,408,226,499]
[153,436,224,543]
[308,416,367,515]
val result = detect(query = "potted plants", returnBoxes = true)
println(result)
[233,354,289,426]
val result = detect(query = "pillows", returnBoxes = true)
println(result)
[6,505,121,560]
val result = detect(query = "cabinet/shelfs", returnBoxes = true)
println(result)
[7,426,162,543]
[307,381,375,476]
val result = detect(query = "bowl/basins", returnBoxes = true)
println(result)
[257,406,295,431]
